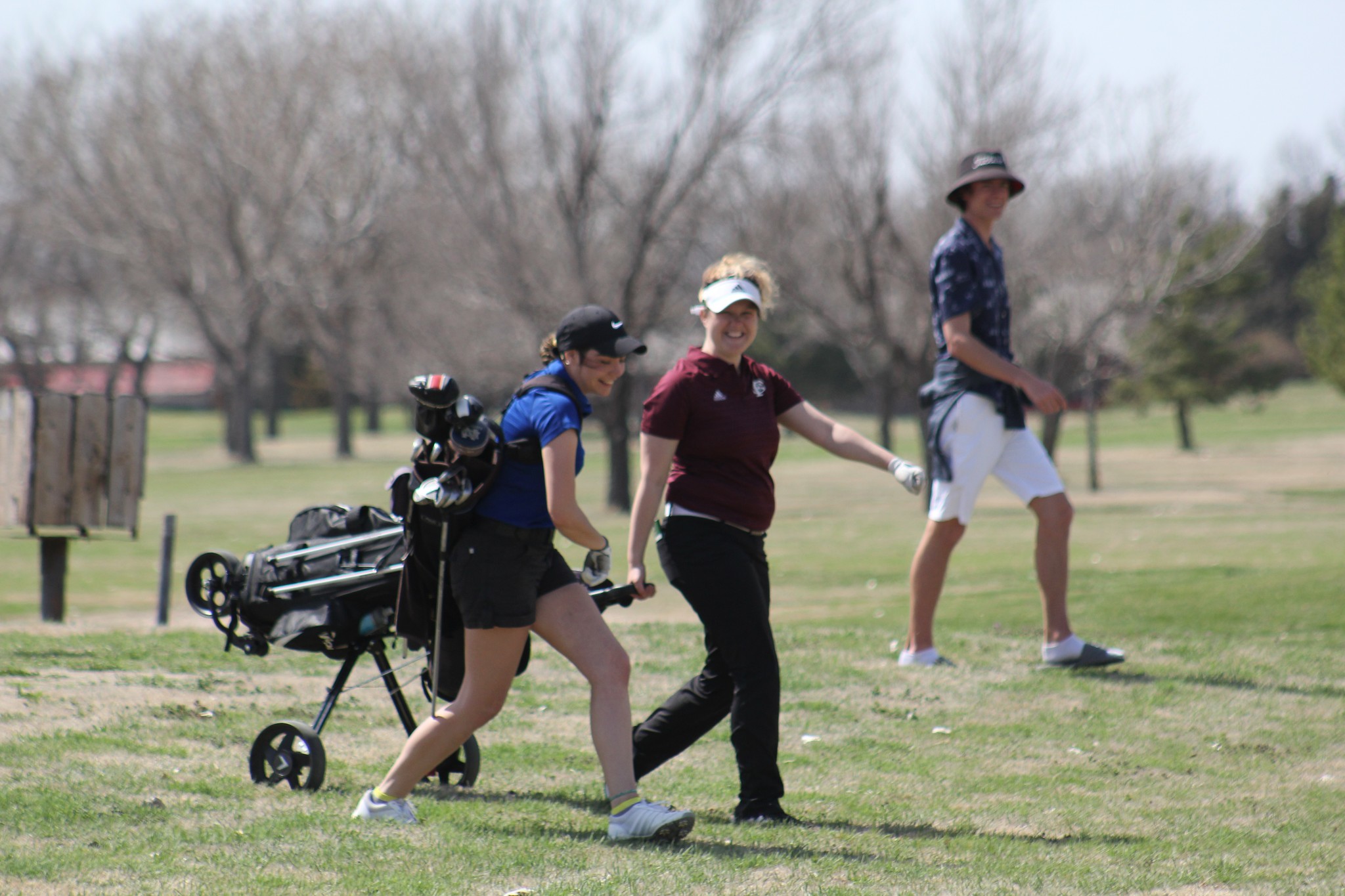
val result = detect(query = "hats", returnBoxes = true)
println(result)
[555,301,647,358]
[700,276,764,319]
[945,151,1025,208]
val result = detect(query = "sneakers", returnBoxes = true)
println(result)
[608,795,695,843]
[351,788,421,825]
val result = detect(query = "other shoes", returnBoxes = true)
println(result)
[734,810,802,829]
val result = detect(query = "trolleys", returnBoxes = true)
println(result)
[184,503,648,792]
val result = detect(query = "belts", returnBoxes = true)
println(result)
[663,503,765,537]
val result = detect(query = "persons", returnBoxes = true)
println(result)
[350,304,694,844]
[892,151,1127,668]
[602,251,924,826]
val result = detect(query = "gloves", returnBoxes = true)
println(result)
[889,457,924,496]
[581,537,611,588]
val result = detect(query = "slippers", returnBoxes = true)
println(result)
[898,657,956,670]
[1041,643,1126,668]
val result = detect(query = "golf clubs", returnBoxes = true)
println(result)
[402,393,488,718]
